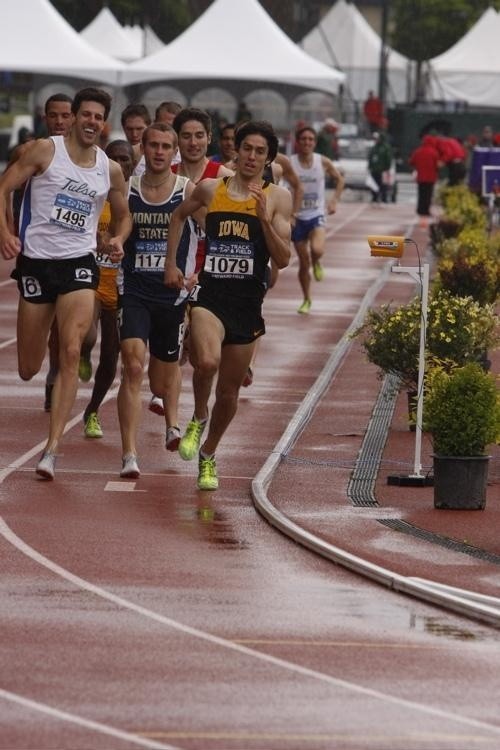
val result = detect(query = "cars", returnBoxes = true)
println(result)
[325,138,397,201]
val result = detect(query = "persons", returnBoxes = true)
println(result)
[465,123,500,203]
[0,88,133,481]
[149,107,253,416]
[131,102,182,176]
[0,94,106,413]
[120,103,152,165]
[278,127,345,315]
[96,123,208,477]
[165,118,292,491]
[205,123,237,163]
[427,129,466,187]
[264,153,305,232]
[7,128,32,237]
[367,132,399,202]
[364,91,383,130]
[78,140,140,439]
[408,133,446,216]
[315,119,341,161]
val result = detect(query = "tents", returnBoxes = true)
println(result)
[0,0,126,174]
[116,0,347,143]
[78,7,166,63]
[297,0,420,113]
[421,6,500,110]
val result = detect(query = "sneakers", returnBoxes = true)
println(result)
[313,262,324,281]
[85,412,104,437]
[78,359,93,381]
[178,418,208,460]
[150,393,165,413]
[35,450,56,480]
[243,368,253,388]
[297,297,313,314]
[120,453,141,478]
[165,426,183,451]
[196,453,220,490]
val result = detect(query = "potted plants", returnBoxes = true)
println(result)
[420,361,500,513]
[346,286,499,437]
